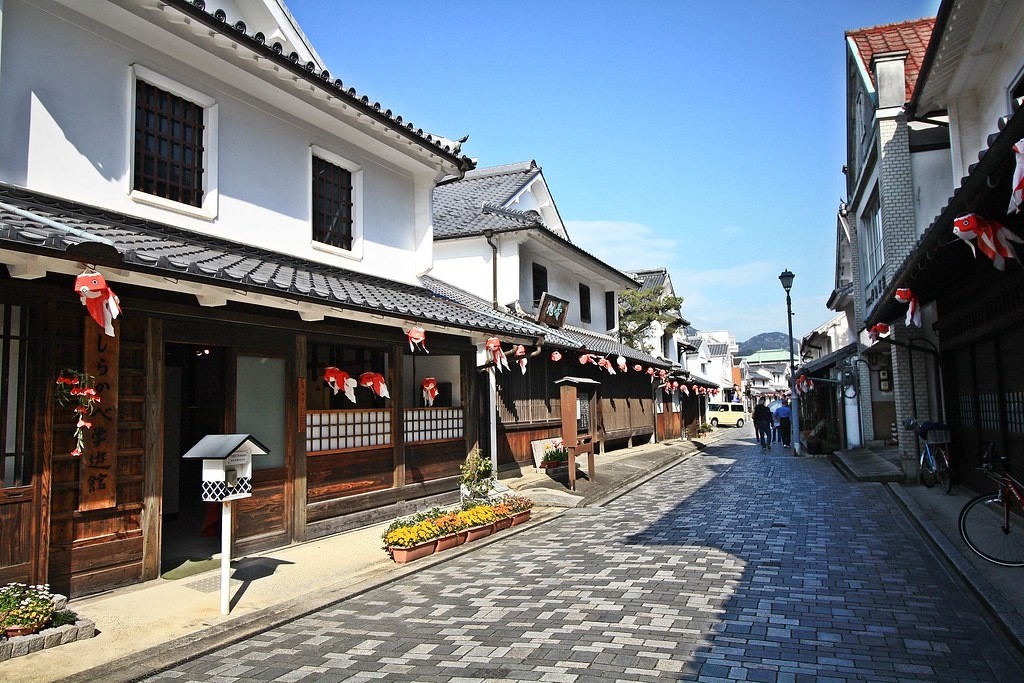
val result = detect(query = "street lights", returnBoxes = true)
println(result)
[777,267,802,457]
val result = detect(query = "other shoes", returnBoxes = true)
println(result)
[763,448,766,451]
[773,441,776,443]
[778,442,781,444]
[783,444,787,448]
[787,445,790,448]
[765,442,771,450]
[757,439,759,442]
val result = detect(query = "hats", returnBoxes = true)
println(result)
[782,400,788,404]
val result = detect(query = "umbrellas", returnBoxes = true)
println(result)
[768,400,783,412]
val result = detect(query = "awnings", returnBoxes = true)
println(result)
[750,386,774,398]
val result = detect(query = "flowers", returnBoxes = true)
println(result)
[56,367,100,457]
[381,494,535,548]
[542,440,568,461]
[0,581,57,635]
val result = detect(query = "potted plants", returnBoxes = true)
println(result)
[697,423,713,438]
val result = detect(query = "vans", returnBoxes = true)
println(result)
[707,401,746,429]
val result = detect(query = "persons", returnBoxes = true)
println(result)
[772,414,782,444]
[773,400,792,447]
[752,397,775,451]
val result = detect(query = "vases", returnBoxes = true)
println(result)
[4,624,33,638]
[393,508,532,564]
[539,461,568,470]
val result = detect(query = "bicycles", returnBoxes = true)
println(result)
[901,417,954,494]
[956,440,1024,567]
